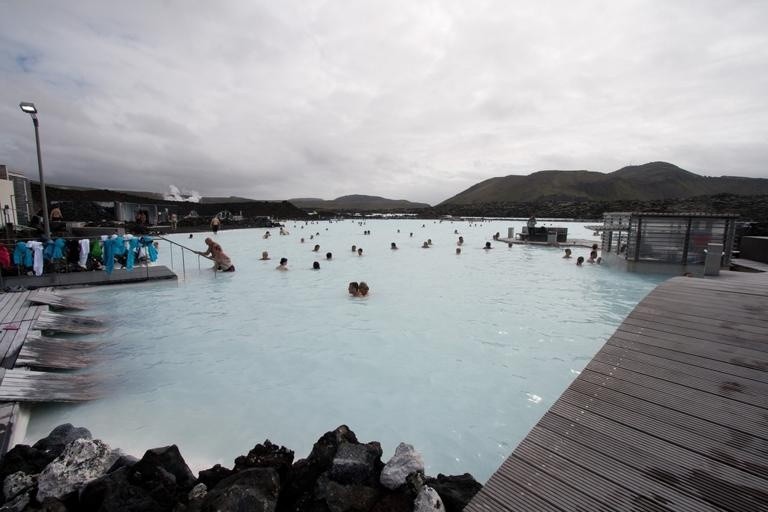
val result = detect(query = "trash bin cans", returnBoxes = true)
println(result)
[704,242,724,276]
[546,231,557,242]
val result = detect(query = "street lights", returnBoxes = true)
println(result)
[21,102,53,240]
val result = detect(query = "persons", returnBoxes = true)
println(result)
[683,272,694,277]
[133,208,604,297]
[50,205,63,220]
